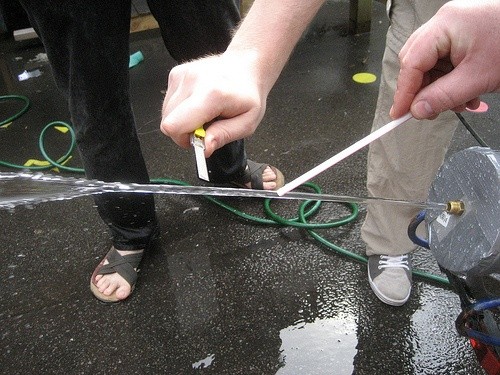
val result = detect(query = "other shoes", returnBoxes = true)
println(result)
[369,252,415,305]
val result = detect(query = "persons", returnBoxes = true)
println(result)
[390,0,500,119]
[17,0,285,303]
[160,0,465,307]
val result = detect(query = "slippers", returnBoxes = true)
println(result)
[233,157,285,189]
[91,243,144,302]
